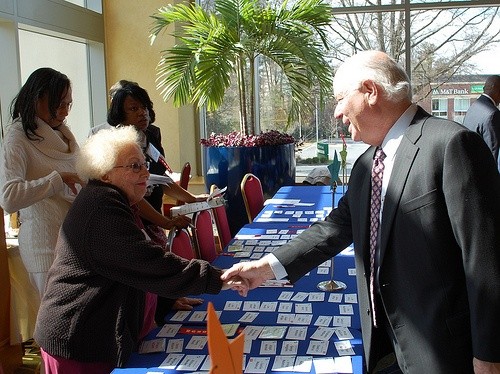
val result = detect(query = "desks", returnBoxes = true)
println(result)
[108,186,364,374]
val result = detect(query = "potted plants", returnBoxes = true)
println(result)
[147,0,335,239]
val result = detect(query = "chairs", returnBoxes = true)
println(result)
[139,163,266,263]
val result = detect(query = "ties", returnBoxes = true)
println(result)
[368,147,388,328]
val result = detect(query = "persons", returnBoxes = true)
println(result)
[219,49,500,374]
[0,68,107,309]
[106,80,213,344]
[462,75,500,175]
[32,124,250,374]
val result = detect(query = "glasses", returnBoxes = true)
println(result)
[59,101,72,110]
[113,161,151,171]
